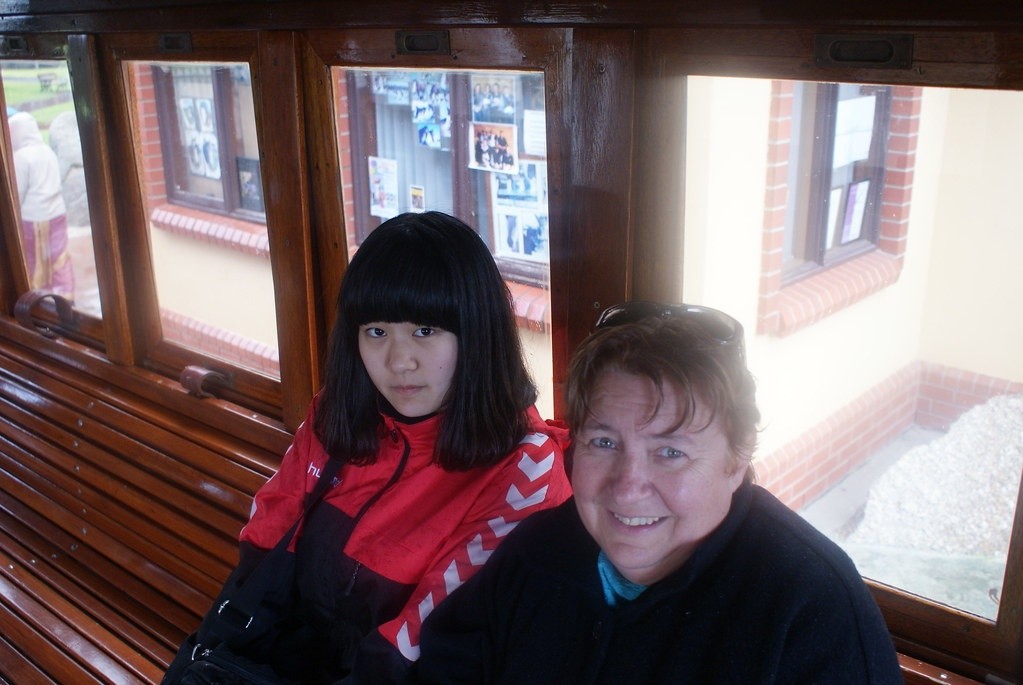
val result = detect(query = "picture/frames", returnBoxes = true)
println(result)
[236,158,264,212]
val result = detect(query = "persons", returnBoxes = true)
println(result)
[186,138,203,174]
[471,81,514,170]
[5,109,75,311]
[367,160,424,212]
[157,210,579,685]
[201,141,221,179]
[197,100,213,133]
[47,109,103,300]
[182,99,197,132]
[414,299,903,685]
[370,70,451,150]
[495,172,549,257]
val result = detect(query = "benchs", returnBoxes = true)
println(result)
[0,308,984,685]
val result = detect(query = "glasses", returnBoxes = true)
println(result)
[595,300,747,386]
